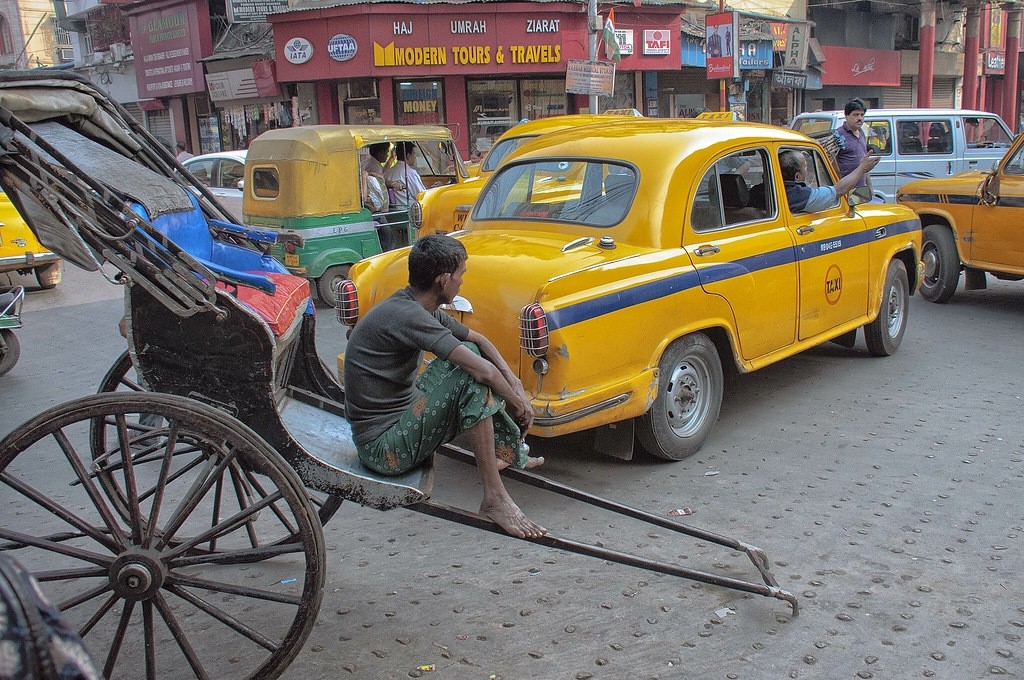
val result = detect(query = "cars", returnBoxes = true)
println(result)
[411,114,639,242]
[334,120,927,464]
[175,150,249,245]
[894,130,1024,304]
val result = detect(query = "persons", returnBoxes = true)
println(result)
[689,107,750,202]
[344,234,548,540]
[945,121,988,153]
[384,141,426,210]
[360,142,397,176]
[749,149,881,213]
[706,24,723,58]
[173,140,194,173]
[832,98,874,196]
[725,27,732,56]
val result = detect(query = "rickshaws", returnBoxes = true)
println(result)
[0,69,798,680]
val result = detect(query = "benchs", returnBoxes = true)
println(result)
[122,185,312,348]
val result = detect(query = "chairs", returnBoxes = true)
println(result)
[900,122,949,153]
[504,173,762,229]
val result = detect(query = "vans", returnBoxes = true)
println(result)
[790,108,1024,206]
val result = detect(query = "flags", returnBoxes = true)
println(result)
[602,7,621,63]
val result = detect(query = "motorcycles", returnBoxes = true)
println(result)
[0,284,25,377]
[241,125,471,308]
[0,186,65,288]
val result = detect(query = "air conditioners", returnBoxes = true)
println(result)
[62,48,74,60]
[93,52,104,65]
[109,43,126,62]
